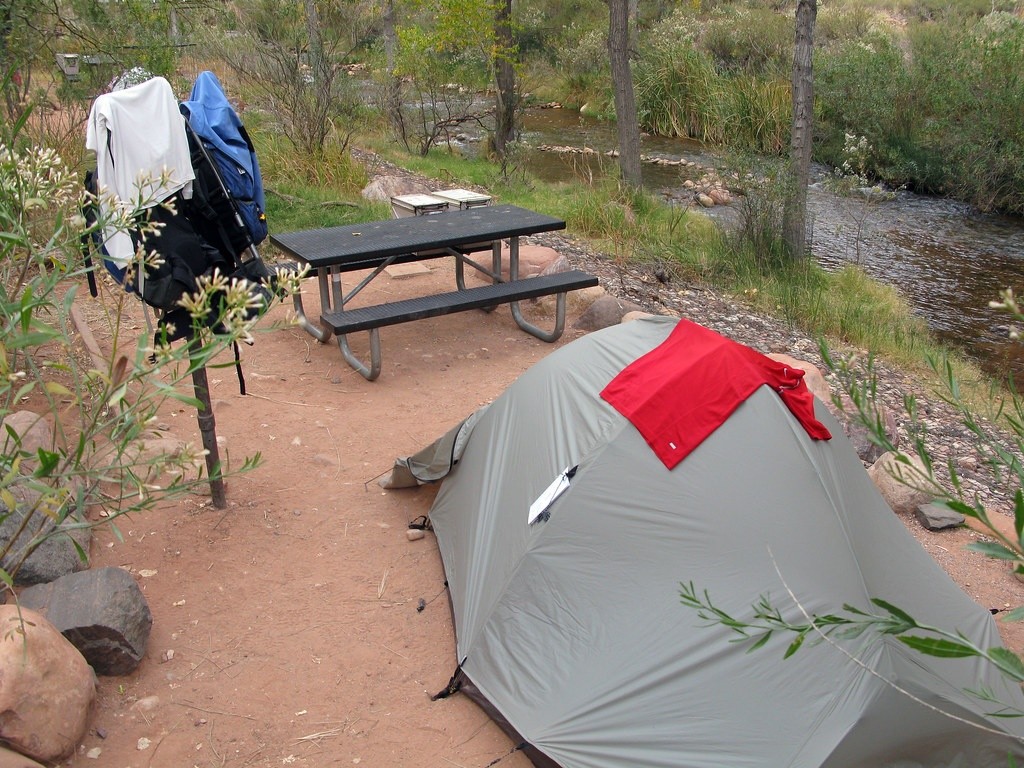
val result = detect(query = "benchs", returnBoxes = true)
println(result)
[265,240,502,323]
[320,269,599,380]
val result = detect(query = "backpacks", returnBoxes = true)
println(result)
[78,172,228,309]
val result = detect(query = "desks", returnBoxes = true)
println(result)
[271,203,567,357]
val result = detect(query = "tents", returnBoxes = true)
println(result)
[364,312,1024,767]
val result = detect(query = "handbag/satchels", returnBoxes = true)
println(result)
[207,257,272,334]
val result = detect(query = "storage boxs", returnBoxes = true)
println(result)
[391,193,449,255]
[431,189,491,249]
